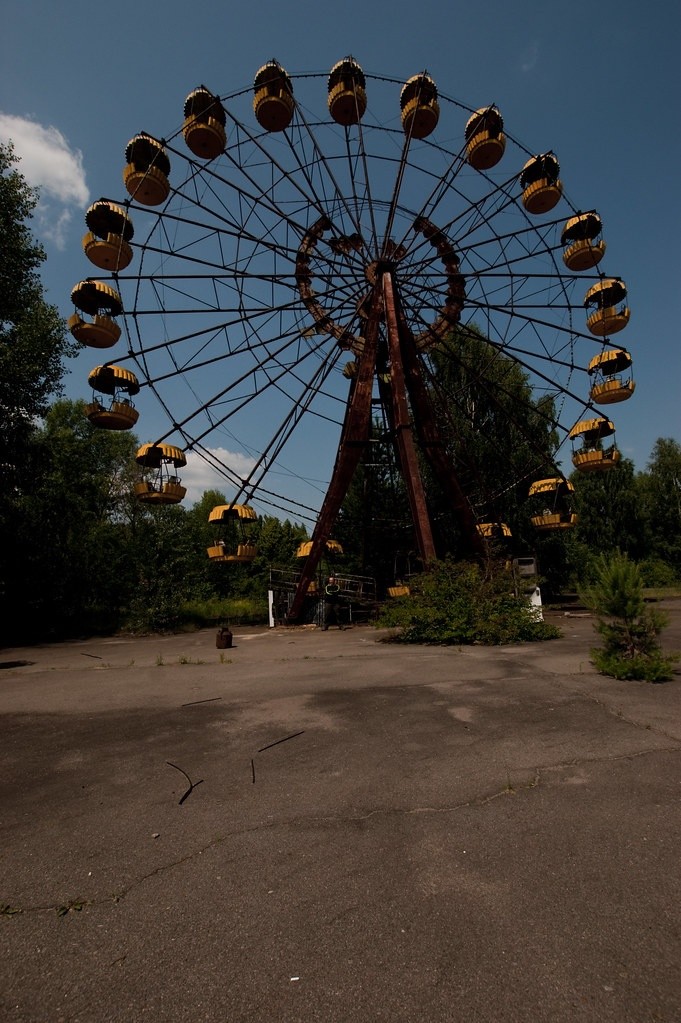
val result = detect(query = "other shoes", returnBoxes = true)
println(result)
[339,626,345,631]
[322,626,327,631]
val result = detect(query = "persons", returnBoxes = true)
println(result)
[321,577,346,631]
